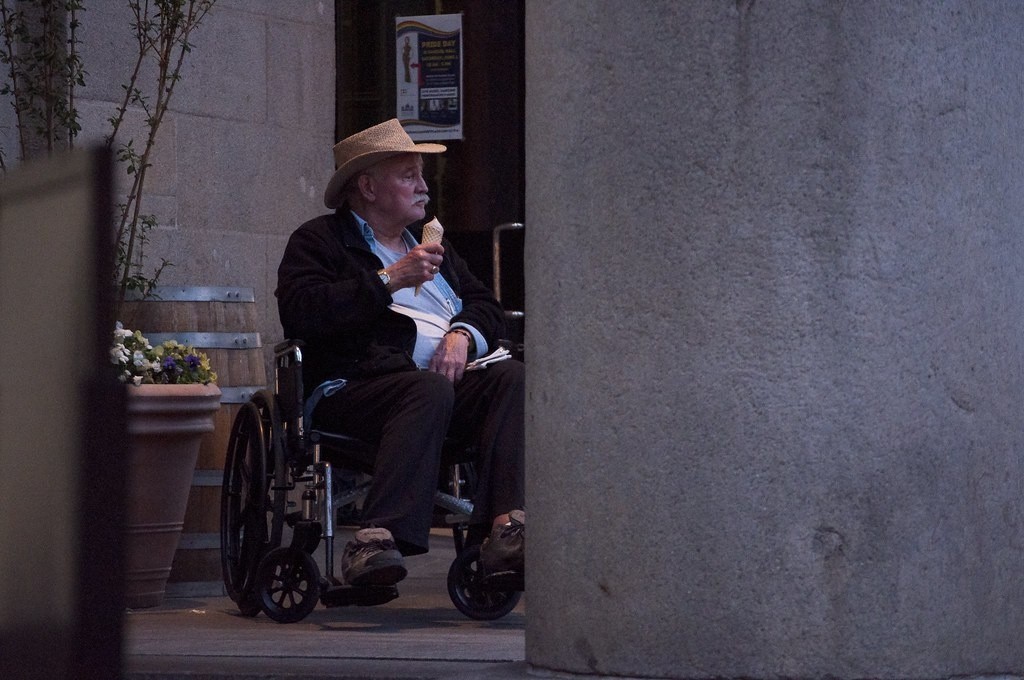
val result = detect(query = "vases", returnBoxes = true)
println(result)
[120,384,221,610]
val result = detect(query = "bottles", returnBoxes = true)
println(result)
[401,104,413,111]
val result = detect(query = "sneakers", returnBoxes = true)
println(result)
[473,510,525,588]
[341,528,408,585]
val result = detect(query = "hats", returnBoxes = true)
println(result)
[324,118,447,209]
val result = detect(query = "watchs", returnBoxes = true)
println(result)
[377,268,393,293]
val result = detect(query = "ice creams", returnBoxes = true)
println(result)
[414,215,444,297]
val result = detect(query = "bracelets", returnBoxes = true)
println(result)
[444,330,472,347]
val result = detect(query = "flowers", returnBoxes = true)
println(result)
[110,321,217,386]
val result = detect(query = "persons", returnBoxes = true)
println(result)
[402,37,411,81]
[277,122,528,587]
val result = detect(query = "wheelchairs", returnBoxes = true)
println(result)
[218,339,526,623]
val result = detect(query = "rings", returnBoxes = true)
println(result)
[431,264,436,274]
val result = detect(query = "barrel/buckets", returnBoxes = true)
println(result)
[116,287,268,599]
